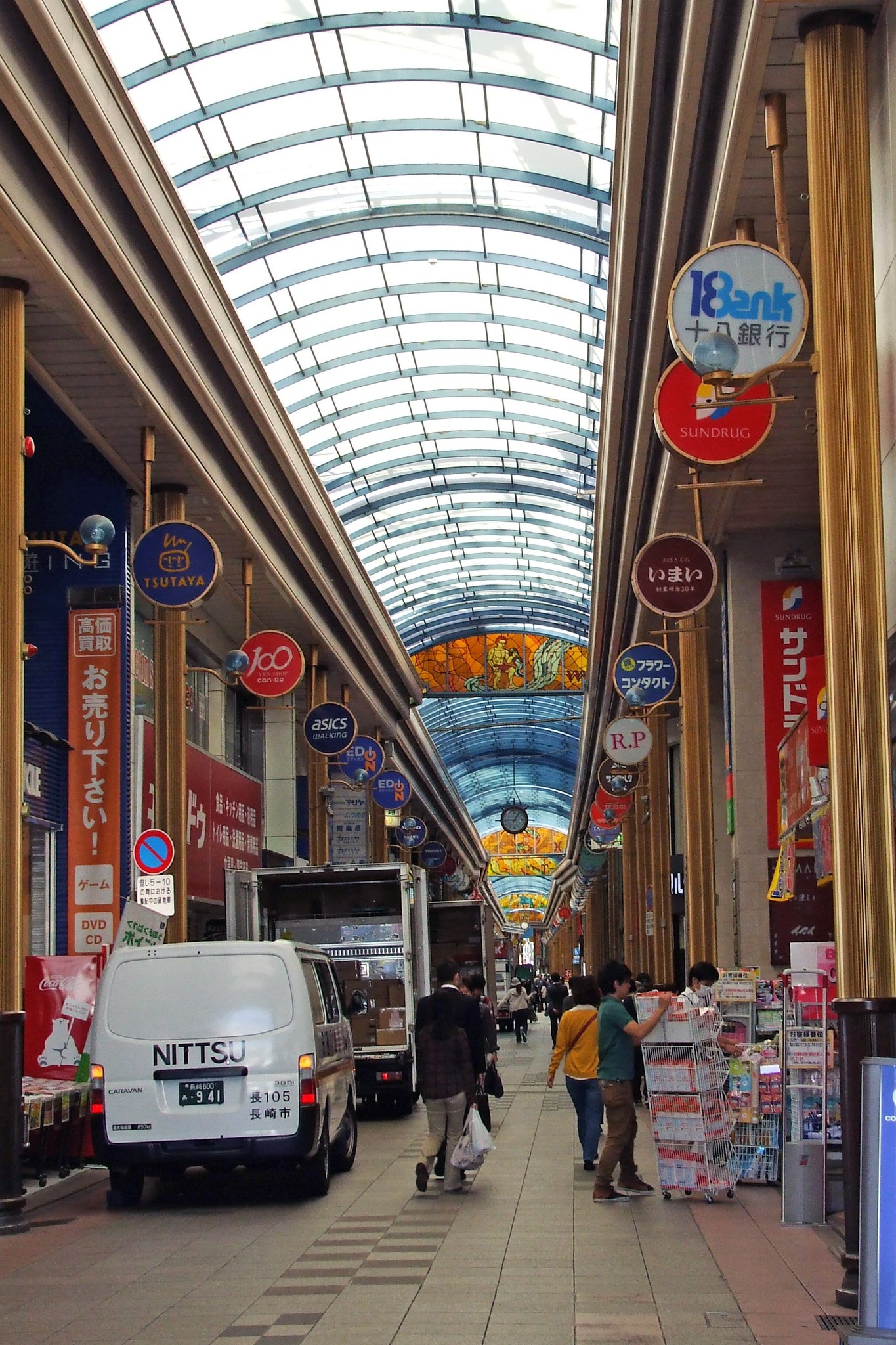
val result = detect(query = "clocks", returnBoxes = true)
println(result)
[495,797,535,839]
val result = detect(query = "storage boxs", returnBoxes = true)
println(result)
[333,958,407,1049]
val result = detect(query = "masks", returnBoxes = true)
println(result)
[692,977,711,998]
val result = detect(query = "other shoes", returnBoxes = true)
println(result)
[460,1173,466,1179]
[415,1162,428,1192]
[445,1186,463,1192]
[584,1152,598,1170]
[434,1157,445,1176]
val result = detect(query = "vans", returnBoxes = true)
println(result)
[89,937,367,1204]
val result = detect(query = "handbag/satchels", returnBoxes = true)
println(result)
[449,1059,504,1170]
[527,995,538,1021]
[549,1000,562,1016]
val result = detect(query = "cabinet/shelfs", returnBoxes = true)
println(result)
[632,964,844,1226]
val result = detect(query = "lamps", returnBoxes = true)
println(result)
[18,514,115,566]
[622,685,683,720]
[691,331,818,409]
[807,774,830,808]
[383,816,416,853]
[568,773,649,917]
[429,870,469,901]
[326,767,368,815]
[183,649,250,688]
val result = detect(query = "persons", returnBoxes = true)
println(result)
[413,961,497,1195]
[680,962,741,1055]
[496,977,537,1043]
[589,961,672,1203]
[536,972,551,1012]
[546,972,569,1050]
[546,982,605,1171]
[559,973,676,1133]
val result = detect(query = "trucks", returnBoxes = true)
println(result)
[425,900,514,1031]
[223,861,432,1120]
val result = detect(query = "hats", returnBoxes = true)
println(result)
[511,977,521,986]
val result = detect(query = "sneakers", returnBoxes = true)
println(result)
[616,1174,655,1196]
[592,1184,630,1203]
